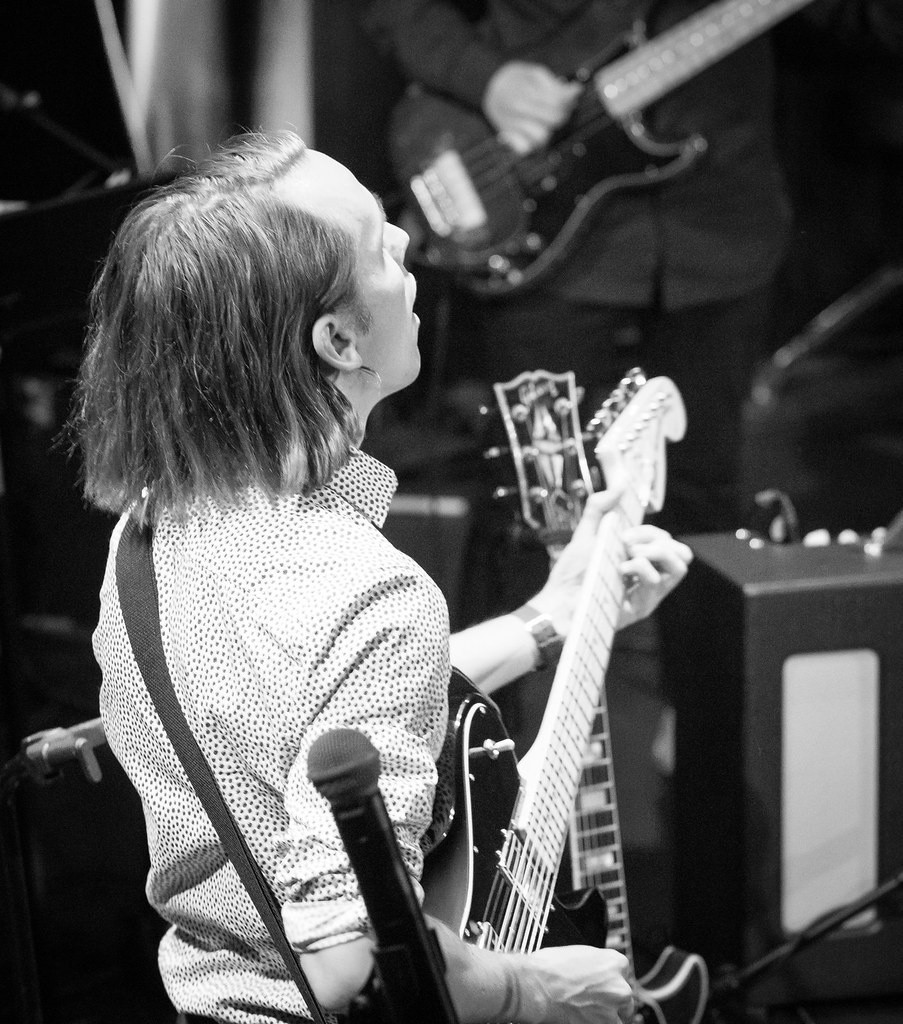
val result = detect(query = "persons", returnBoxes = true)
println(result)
[80,130,694,1024]
[385,0,796,535]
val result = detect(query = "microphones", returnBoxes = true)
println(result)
[309,730,461,1024]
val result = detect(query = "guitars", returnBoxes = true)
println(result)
[382,0,815,301]
[334,362,613,1024]
[477,369,708,1024]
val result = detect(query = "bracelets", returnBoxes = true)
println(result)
[512,604,563,672]
